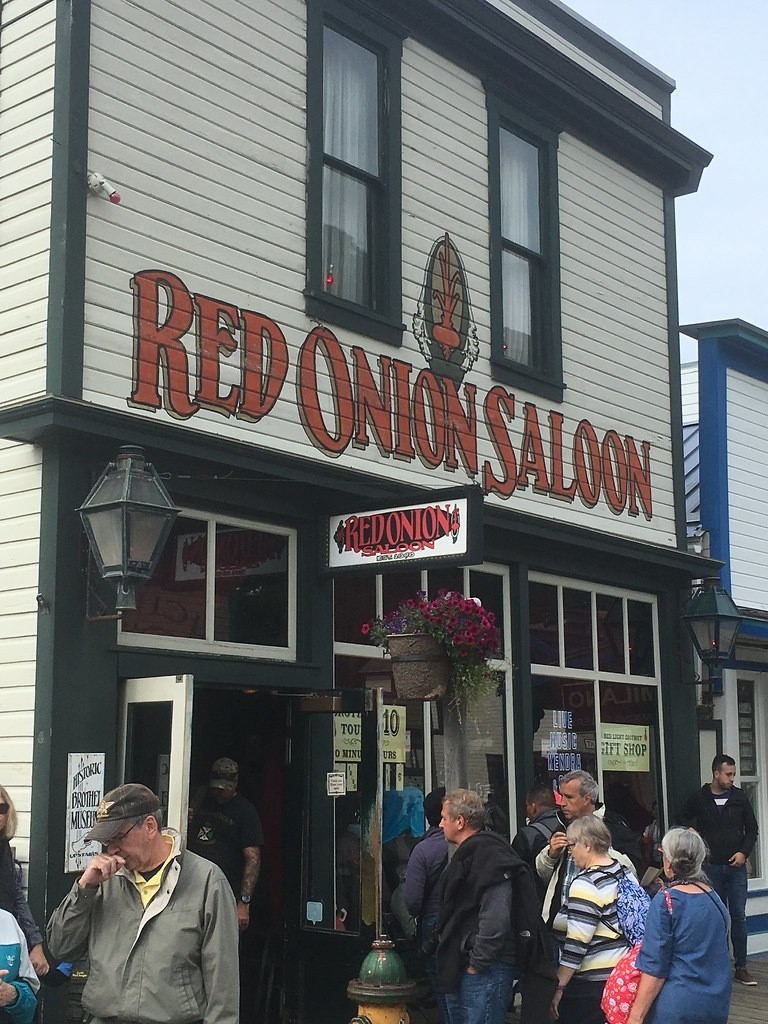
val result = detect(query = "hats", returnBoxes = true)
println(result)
[84,782,161,839]
[208,757,238,789]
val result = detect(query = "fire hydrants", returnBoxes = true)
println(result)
[346,934,420,1024]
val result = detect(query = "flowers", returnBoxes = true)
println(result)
[362,588,518,734]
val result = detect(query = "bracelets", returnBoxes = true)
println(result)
[557,985,566,990]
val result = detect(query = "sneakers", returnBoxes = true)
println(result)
[734,967,758,986]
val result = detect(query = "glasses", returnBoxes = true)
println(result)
[100,814,154,848]
[0,803,10,814]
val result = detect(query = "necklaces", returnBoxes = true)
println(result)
[427,789,516,1024]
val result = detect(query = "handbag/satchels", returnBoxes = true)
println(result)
[600,890,672,1024]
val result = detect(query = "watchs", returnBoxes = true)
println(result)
[236,894,252,907]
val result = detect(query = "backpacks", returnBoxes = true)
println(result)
[587,865,652,943]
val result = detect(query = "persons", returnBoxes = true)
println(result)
[627,828,733,1024]
[406,786,450,1024]
[1,786,47,974]
[49,785,240,1024]
[549,815,639,1023]
[485,793,507,837]
[514,788,559,864]
[187,757,264,930]
[0,909,40,1024]
[521,770,634,1024]
[643,804,664,868]
[689,754,757,985]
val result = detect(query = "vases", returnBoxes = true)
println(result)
[386,633,450,700]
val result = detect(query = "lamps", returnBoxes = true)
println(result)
[74,445,183,620]
[606,597,651,673]
[676,577,743,697]
